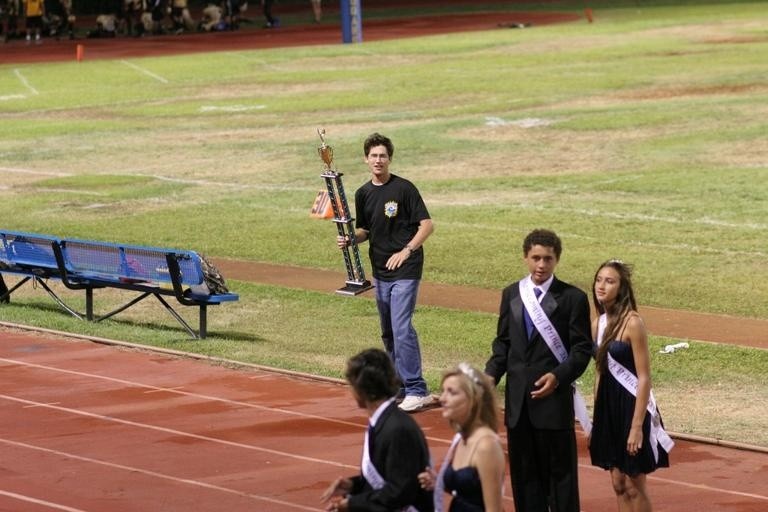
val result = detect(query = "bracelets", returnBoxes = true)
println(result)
[404,245,414,253]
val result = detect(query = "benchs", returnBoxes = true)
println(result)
[1,231,239,343]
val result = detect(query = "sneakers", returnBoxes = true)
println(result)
[397,393,433,412]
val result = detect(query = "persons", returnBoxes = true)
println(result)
[418,362,504,512]
[482,229,591,512]
[321,348,434,512]
[0,0,253,46]
[311,0,322,24]
[589,260,675,512]
[260,0,282,29]
[336,132,434,412]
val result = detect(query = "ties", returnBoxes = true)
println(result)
[369,424,373,454]
[524,288,542,342]
